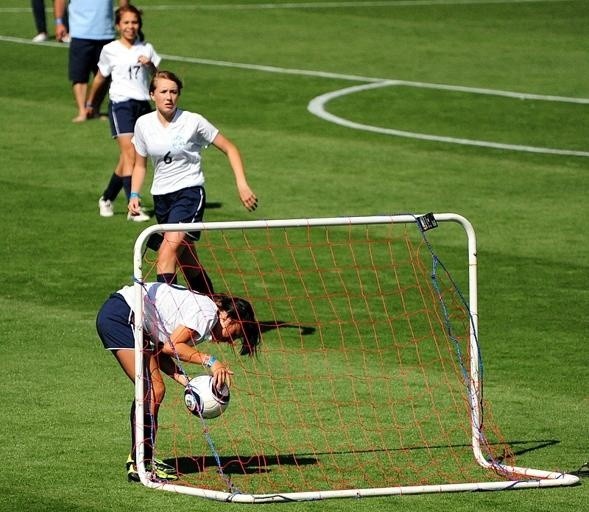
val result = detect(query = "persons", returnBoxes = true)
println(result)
[95,281,254,482]
[53,0,131,122]
[31,0,70,43]
[127,69,259,294]
[82,4,163,222]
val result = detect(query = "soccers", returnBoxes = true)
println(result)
[183,375,230,420]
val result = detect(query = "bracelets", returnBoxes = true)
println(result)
[54,18,65,24]
[201,354,217,368]
[129,192,141,198]
[86,103,94,108]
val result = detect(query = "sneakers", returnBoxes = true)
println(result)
[32,33,49,42]
[126,453,177,474]
[97,196,115,216]
[127,464,178,483]
[127,210,150,222]
[61,33,72,43]
